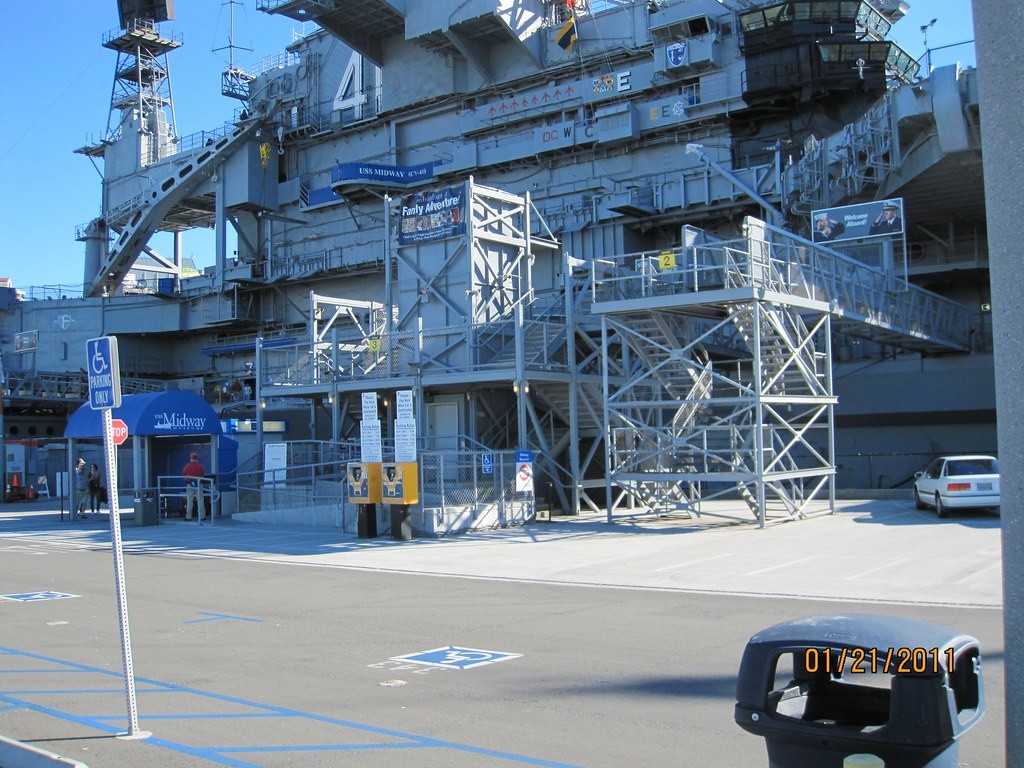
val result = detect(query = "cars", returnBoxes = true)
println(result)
[913,455,999,518]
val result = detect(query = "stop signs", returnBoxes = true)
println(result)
[112,419,128,445]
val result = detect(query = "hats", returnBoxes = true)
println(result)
[881,201,900,211]
[814,212,828,220]
[190,453,198,458]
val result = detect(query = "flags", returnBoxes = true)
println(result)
[565,0,577,12]
[555,16,578,55]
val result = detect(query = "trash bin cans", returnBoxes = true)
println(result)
[133,489,158,526]
[734,611,989,768]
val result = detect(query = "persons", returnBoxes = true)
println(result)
[240,109,248,120]
[869,201,902,235]
[87,464,103,515]
[75,458,89,519]
[214,377,253,406]
[182,452,208,521]
[812,212,845,243]
[238,257,247,267]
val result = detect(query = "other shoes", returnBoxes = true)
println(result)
[82,514,88,518]
[184,518,191,521]
[201,518,206,520]
[97,509,101,515]
[91,512,95,514]
